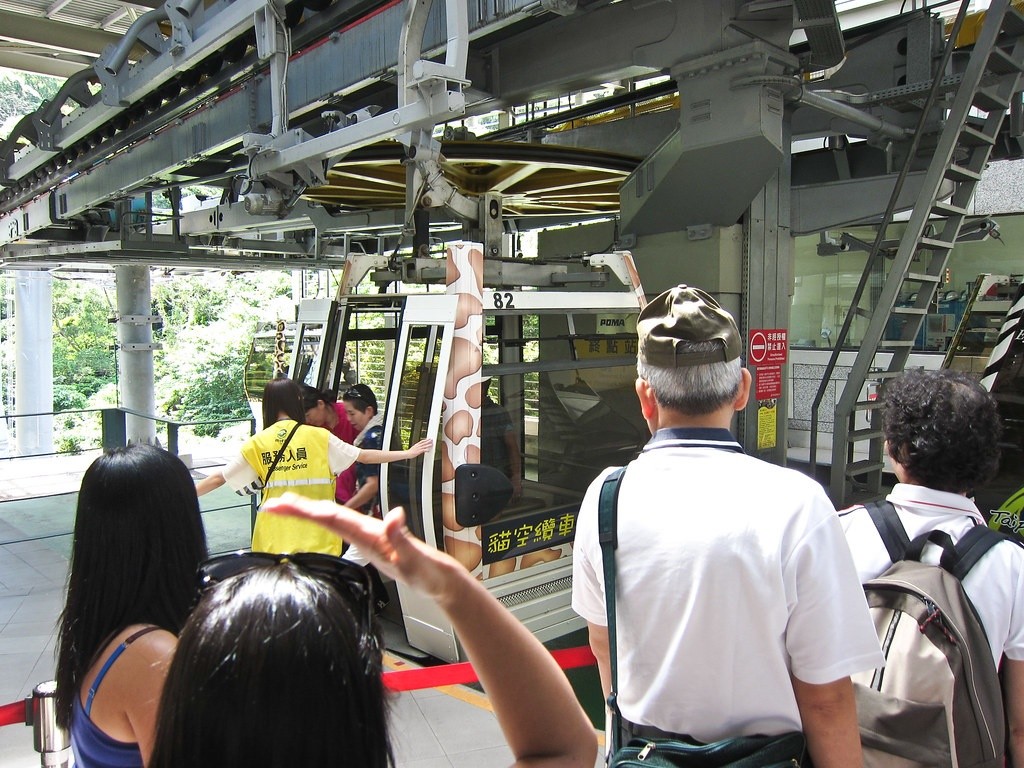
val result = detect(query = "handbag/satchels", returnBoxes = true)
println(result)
[607,730,811,768]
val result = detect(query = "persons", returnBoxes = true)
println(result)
[300,386,392,618]
[194,378,434,557]
[54,443,210,768]
[572,283,887,768]
[150,491,600,768]
[341,384,410,567]
[836,366,1024,767]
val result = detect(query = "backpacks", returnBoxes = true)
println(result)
[849,494,1013,768]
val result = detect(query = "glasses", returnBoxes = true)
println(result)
[343,387,362,400]
[191,551,377,643]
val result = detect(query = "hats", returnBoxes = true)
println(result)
[636,285,744,369]
